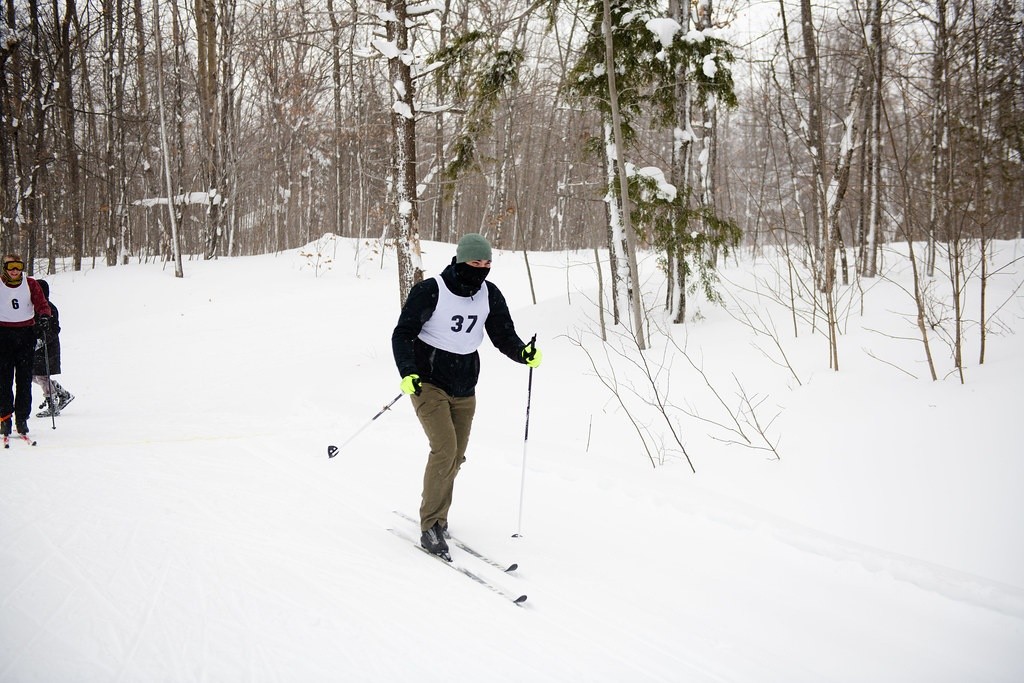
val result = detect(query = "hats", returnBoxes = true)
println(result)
[455,233,492,264]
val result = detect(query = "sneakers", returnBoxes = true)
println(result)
[420,524,448,554]
[443,527,451,539]
[58,391,74,409]
[16,416,29,434]
[0,418,12,436]
[36,400,60,417]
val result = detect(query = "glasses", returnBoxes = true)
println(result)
[2,259,24,270]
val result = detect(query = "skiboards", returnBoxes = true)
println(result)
[3,429,37,448]
[386,510,528,603]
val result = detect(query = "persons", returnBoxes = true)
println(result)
[391,234,543,563]
[32,279,75,418]
[0,254,51,436]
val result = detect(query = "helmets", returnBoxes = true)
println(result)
[36,279,49,298]
[1,255,24,268]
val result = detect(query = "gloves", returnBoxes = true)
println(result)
[33,339,43,351]
[399,374,423,396]
[34,317,49,331]
[521,346,543,368]
